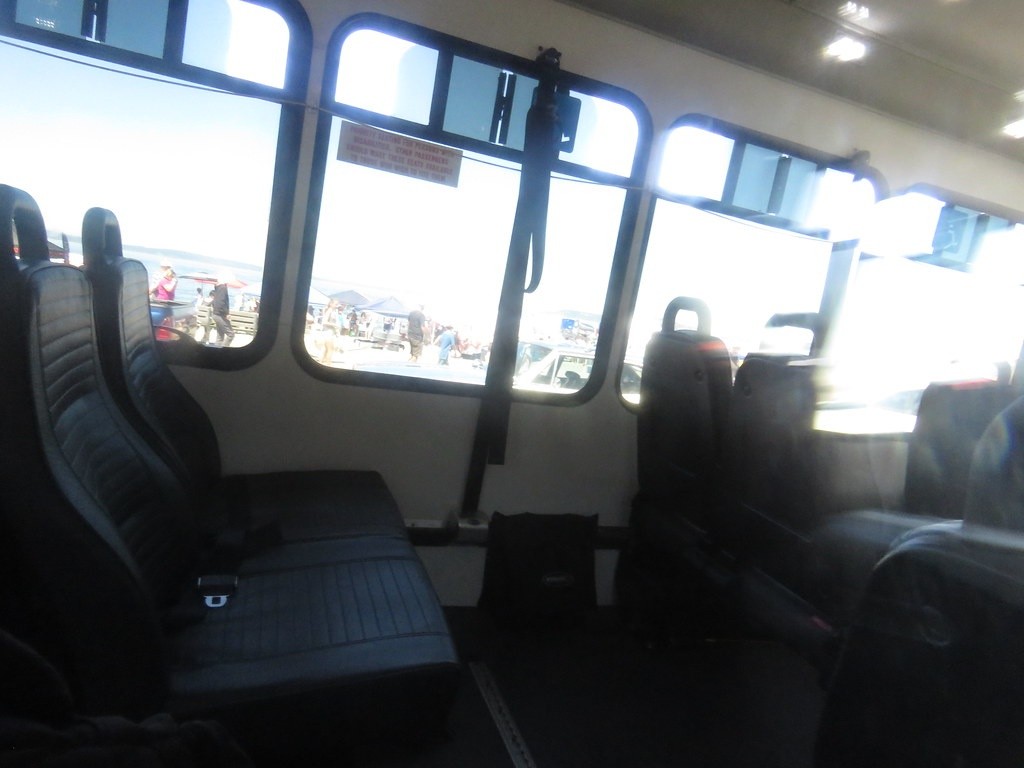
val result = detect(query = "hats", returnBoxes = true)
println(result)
[215,270,233,287]
[158,258,173,267]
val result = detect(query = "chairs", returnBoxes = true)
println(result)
[812,394,1024,768]
[0,184,463,752]
[729,306,896,629]
[635,294,736,550]
[81,205,419,579]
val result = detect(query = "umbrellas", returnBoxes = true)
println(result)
[309,282,465,326]
[180,264,264,296]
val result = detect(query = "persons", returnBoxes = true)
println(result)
[149,263,179,339]
[305,298,492,369]
[190,278,261,348]
